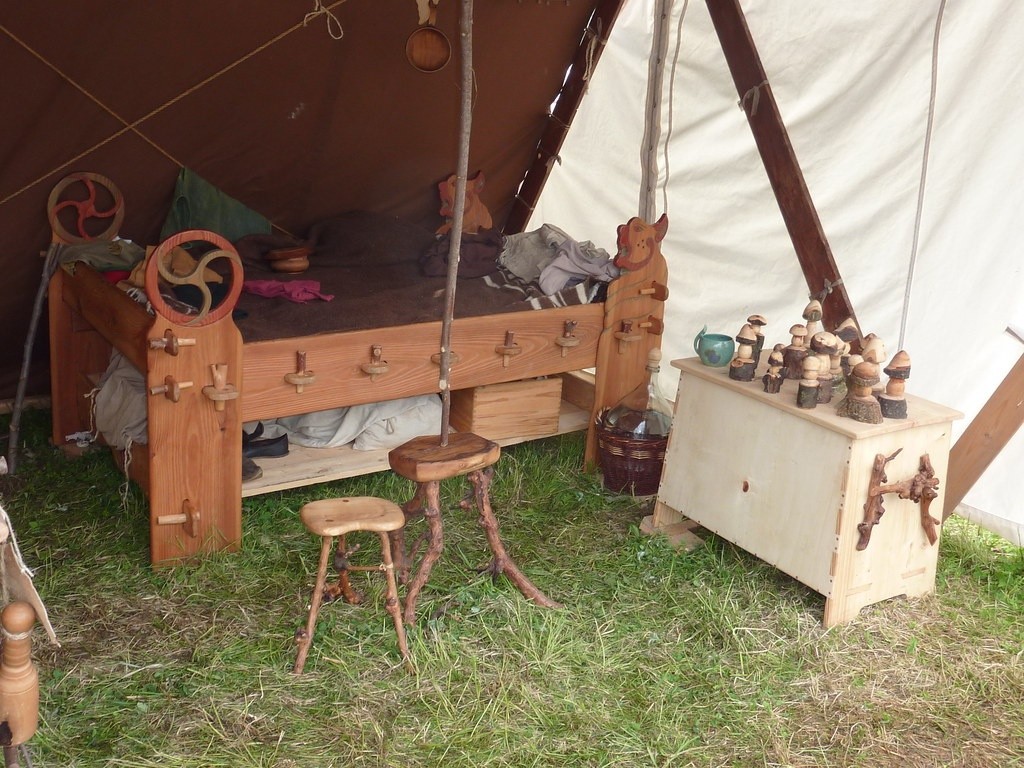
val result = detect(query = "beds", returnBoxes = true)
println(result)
[41,168,672,568]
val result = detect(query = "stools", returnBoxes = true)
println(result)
[298,495,419,679]
[390,431,567,627]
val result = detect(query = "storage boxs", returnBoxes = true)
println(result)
[447,374,563,434]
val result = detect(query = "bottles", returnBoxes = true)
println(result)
[604,347,673,435]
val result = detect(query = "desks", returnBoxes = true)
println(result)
[654,347,966,622]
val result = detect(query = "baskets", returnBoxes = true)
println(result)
[594,405,669,501]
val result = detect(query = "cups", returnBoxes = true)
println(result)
[695,325,735,367]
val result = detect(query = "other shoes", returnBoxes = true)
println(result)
[243,421,289,458]
[243,457,263,482]
